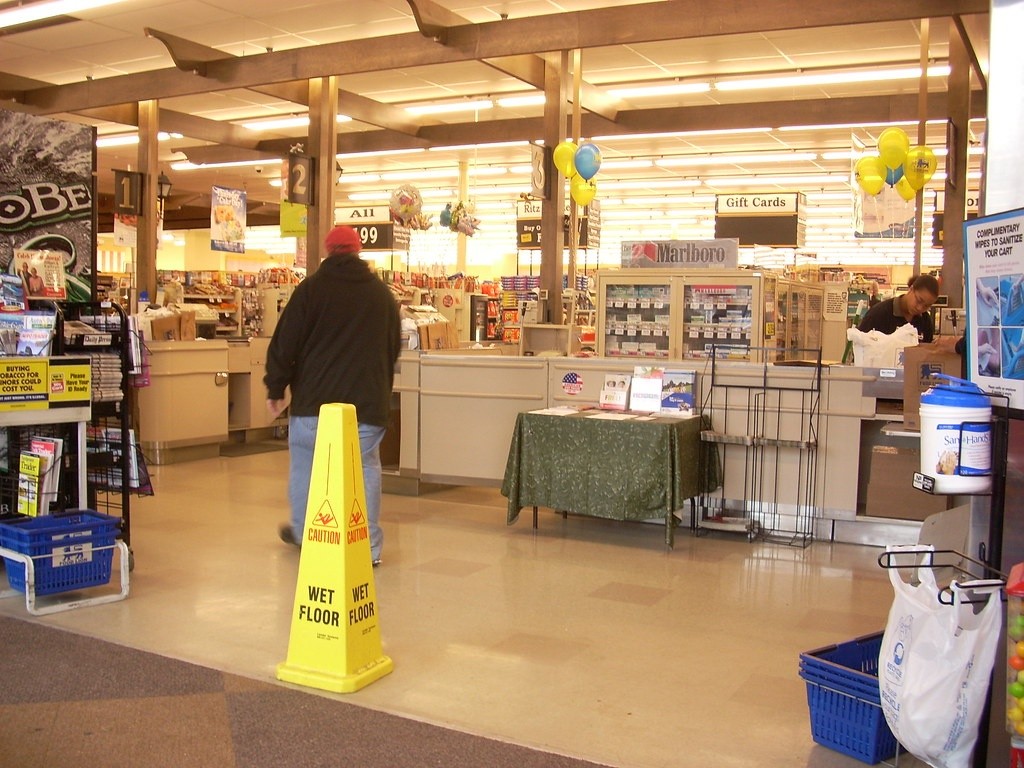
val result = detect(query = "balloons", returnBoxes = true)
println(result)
[854,157,887,197]
[553,141,579,177]
[388,184,432,231]
[885,167,904,188]
[574,143,601,183]
[896,175,918,202]
[440,201,481,236]
[878,127,909,169]
[902,147,936,191]
[569,172,597,208]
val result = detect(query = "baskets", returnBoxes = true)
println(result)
[0,506,121,597]
[799,630,908,764]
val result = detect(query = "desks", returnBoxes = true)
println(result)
[501,407,722,544]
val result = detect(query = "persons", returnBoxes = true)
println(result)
[856,274,940,342]
[936,450,957,475]
[262,223,409,567]
[679,402,688,411]
[18,262,32,287]
[679,384,685,392]
[977,277,1024,376]
[934,325,966,378]
[29,268,44,292]
[667,385,675,394]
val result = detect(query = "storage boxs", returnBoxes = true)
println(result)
[904,344,964,431]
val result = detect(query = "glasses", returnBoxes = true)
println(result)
[913,286,932,311]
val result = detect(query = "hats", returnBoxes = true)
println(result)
[322,226,362,255]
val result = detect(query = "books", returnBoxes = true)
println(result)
[0,249,150,519]
[598,364,698,416]
[700,517,759,531]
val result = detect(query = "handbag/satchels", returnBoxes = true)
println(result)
[880,543,1002,767]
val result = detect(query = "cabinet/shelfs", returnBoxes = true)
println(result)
[98,268,825,359]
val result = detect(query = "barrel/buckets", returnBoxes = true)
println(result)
[919,373,993,493]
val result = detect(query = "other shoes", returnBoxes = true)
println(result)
[371,558,381,565]
[279,524,302,548]
